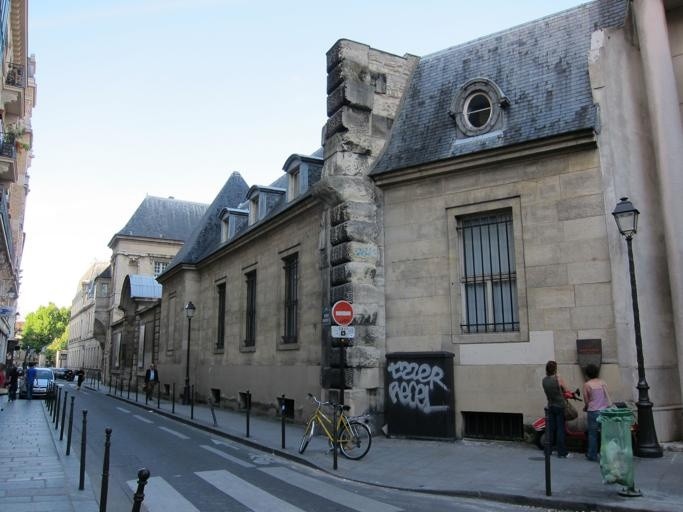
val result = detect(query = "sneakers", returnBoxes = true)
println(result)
[559,452,576,459]
[586,453,595,460]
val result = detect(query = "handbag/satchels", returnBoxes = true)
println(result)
[602,383,617,408]
[565,401,578,421]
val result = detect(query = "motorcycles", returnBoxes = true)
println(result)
[528,387,639,456]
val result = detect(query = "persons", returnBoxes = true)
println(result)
[143,363,160,401]
[0,358,7,387]
[74,365,85,391]
[24,360,37,400]
[541,359,579,460]
[582,364,612,461]
[8,365,19,401]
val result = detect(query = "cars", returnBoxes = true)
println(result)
[18,368,55,399]
[56,368,69,379]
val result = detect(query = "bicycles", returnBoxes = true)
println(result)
[297,393,372,461]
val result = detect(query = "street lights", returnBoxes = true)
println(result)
[182,301,195,404]
[612,195,664,459]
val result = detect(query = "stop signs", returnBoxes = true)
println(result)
[332,300,355,326]
